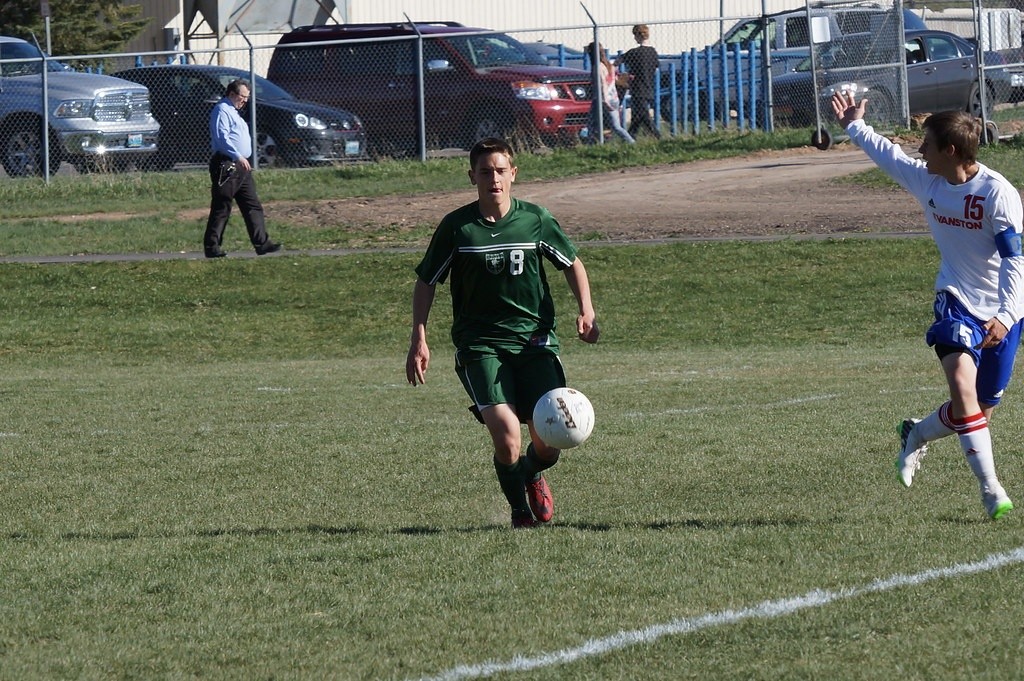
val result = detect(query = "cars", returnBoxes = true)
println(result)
[106,63,370,172]
[771,28,1014,131]
[479,36,607,71]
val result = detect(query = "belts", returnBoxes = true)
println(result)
[216,150,238,164]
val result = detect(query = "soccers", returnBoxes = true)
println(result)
[533,387,595,450]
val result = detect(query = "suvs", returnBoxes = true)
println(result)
[0,35,162,180]
[656,0,936,128]
[268,20,612,160]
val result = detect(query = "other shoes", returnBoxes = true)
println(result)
[256,243,282,255]
[206,251,227,258]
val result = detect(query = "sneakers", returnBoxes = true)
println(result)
[510,503,534,529]
[980,482,1014,520]
[896,417,929,489]
[520,455,554,523]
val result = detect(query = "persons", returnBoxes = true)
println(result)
[406,138,602,527]
[201,79,280,258]
[829,88,1024,520]
[587,43,635,145]
[614,25,663,139]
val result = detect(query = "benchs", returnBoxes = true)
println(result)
[929,44,957,57]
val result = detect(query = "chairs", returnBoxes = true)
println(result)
[908,49,925,64]
